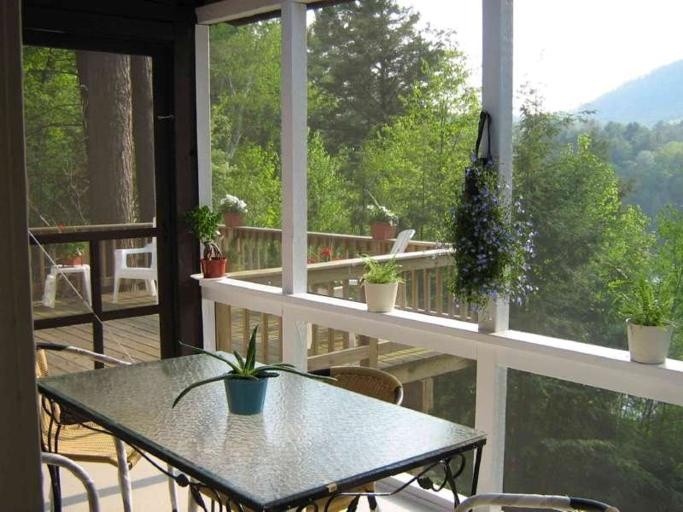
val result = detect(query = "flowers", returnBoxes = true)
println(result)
[217,193,249,215]
[366,204,399,227]
[426,152,542,316]
[57,223,89,260]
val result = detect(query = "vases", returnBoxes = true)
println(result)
[223,212,242,227]
[372,223,395,241]
[62,256,83,268]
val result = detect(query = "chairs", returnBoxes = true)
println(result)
[34,341,180,512]
[453,493,621,512]
[187,366,404,512]
[112,216,159,305]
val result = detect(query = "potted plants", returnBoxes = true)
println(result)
[599,265,683,364]
[171,324,338,415]
[176,204,228,278]
[359,253,407,313]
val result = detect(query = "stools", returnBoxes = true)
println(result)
[42,263,92,310]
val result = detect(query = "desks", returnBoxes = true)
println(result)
[34,350,489,512]
